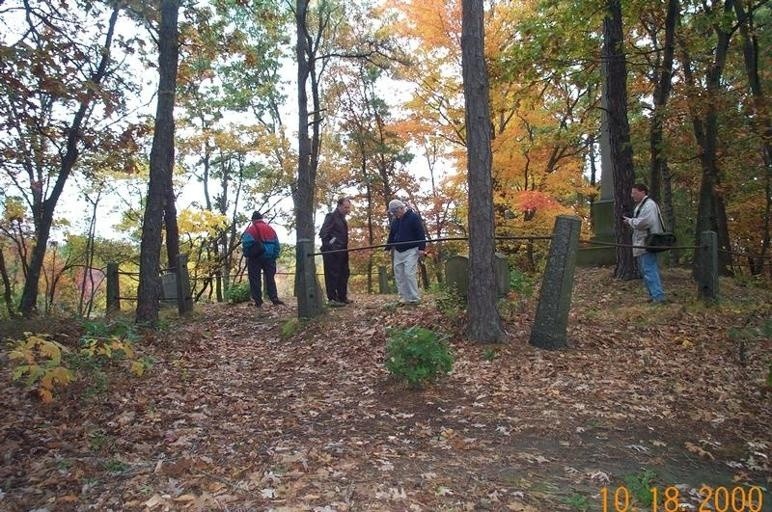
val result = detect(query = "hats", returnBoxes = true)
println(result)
[251,210,263,220]
[387,199,406,213]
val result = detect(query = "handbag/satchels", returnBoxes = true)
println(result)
[418,256,430,290]
[242,223,266,258]
[635,196,678,253]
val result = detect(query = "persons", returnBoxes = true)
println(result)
[242,210,287,306]
[318,197,354,309]
[620,183,670,304]
[384,199,428,306]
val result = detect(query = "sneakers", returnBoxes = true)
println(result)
[399,298,421,304]
[328,296,354,306]
[255,299,285,307]
